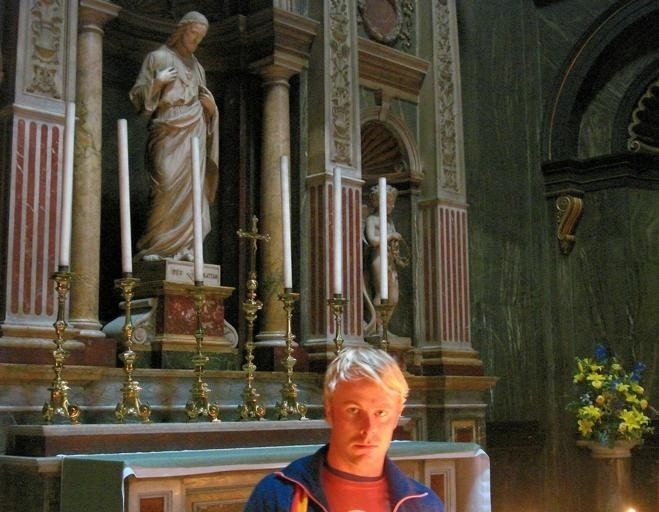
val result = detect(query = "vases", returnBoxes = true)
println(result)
[577,435,639,459]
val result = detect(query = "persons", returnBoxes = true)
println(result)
[129,11,221,262]
[366,183,403,327]
[243,345,446,512]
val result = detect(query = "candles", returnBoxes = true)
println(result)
[376,175,388,298]
[55,99,78,272]
[187,134,206,284]
[116,115,137,275]
[329,166,344,296]
[278,153,296,291]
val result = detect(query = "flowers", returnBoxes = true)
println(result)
[567,344,654,443]
